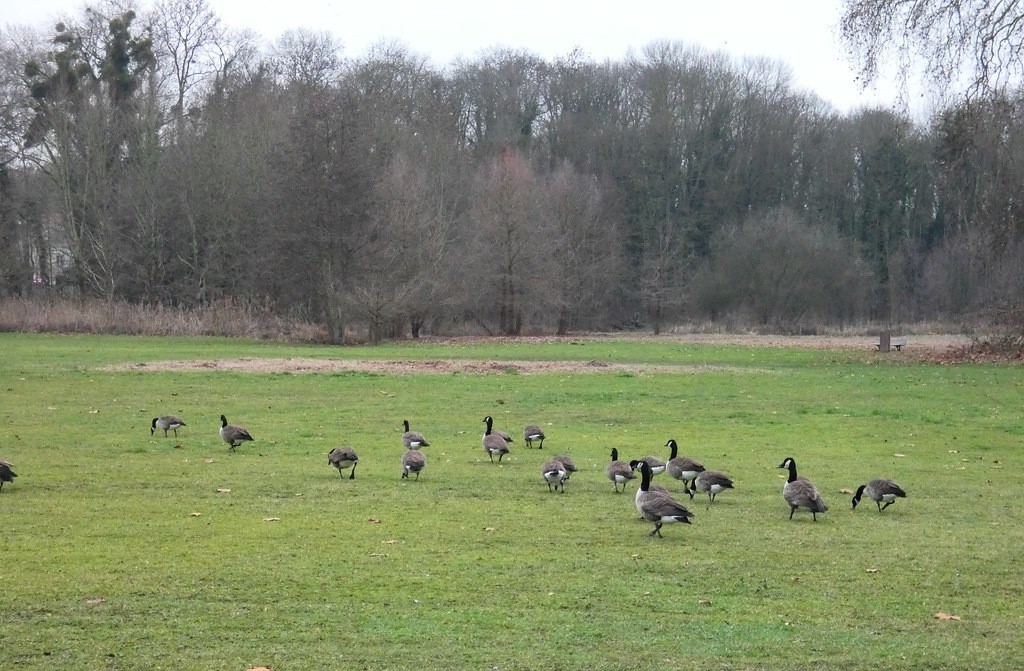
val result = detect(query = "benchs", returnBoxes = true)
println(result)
[871,337,907,351]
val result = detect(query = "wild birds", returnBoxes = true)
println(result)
[0,459,19,492]
[605,438,735,539]
[481,416,514,465]
[151,415,188,439]
[850,477,908,514]
[541,453,579,495]
[327,446,359,480]
[523,424,546,450]
[400,420,431,481]
[219,414,255,453]
[775,457,828,523]
